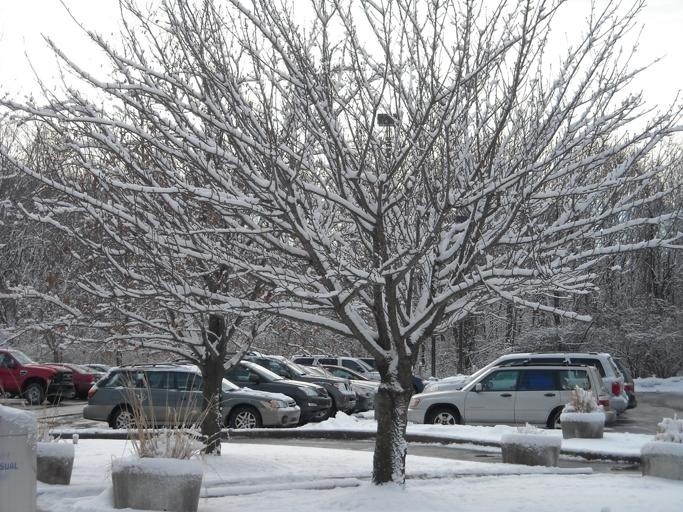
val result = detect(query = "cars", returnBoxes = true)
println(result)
[201,356,425,422]
[1,348,111,405]
[82,361,301,427]
[407,351,637,428]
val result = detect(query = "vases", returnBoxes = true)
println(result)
[36,443,201,512]
[502,412,682,481]
